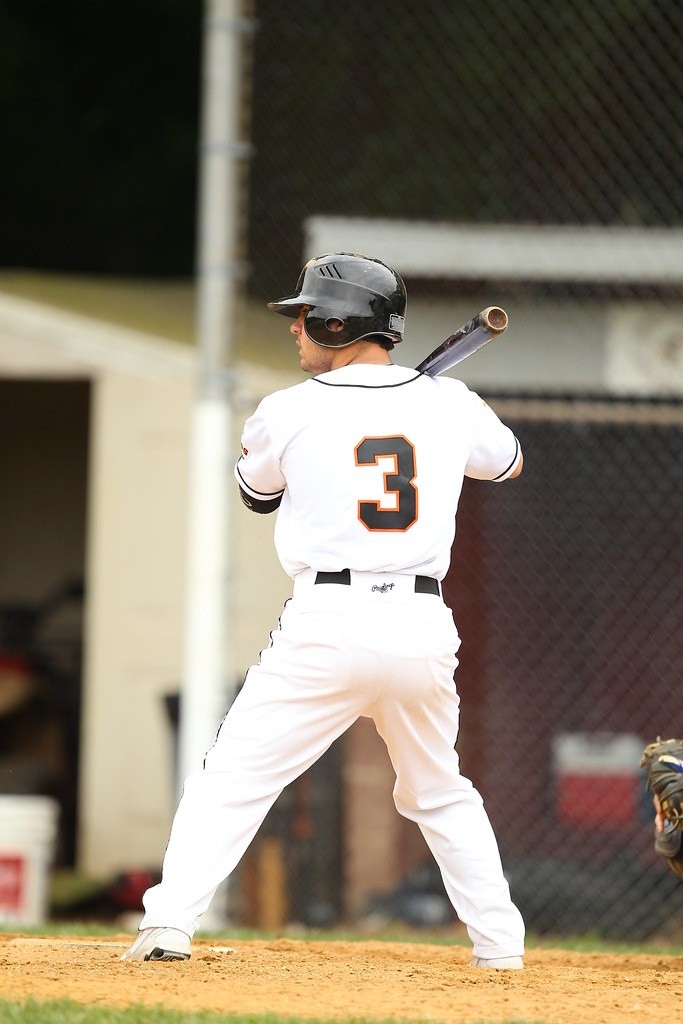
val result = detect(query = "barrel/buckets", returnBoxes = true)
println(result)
[0,793,62,927]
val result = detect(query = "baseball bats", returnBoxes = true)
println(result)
[413,304,509,378]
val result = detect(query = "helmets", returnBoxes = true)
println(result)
[267,252,407,348]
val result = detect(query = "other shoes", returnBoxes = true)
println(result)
[467,957,524,971]
[119,926,191,961]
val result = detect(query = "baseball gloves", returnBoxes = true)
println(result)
[637,735,683,878]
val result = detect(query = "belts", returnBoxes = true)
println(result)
[314,570,441,596]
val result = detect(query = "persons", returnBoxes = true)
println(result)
[113,253,526,971]
